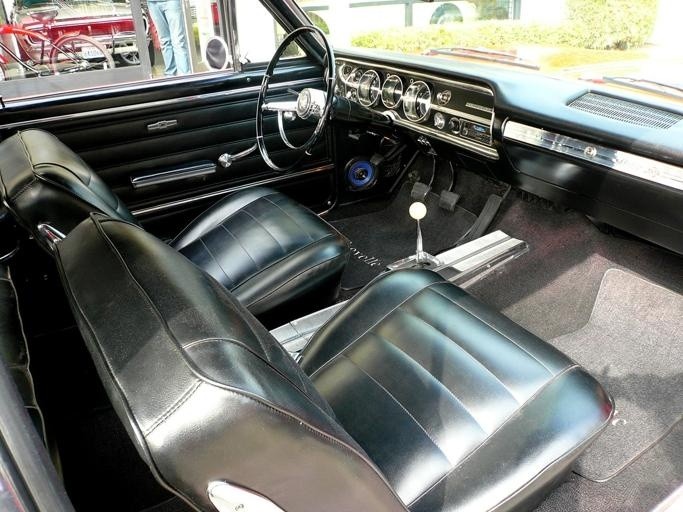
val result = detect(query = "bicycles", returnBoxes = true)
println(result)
[0,6,116,81]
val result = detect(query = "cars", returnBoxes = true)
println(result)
[331,0,476,39]
[10,1,153,67]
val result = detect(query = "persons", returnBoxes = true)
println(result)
[147,0,190,78]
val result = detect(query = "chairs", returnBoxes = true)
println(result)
[1,129,347,311]
[55,211,615,511]
